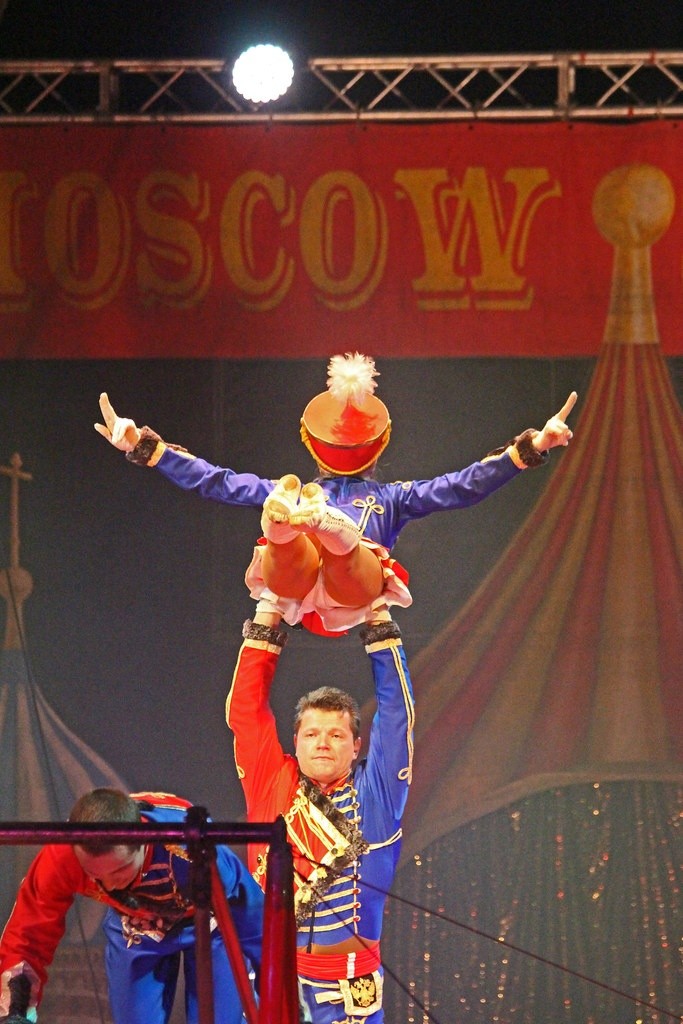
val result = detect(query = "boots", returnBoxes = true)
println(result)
[261,474,300,544]
[289,482,361,555]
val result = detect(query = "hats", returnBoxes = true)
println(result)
[300,350,392,476]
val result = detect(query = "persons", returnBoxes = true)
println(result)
[90,384,582,639]
[0,787,269,1023]
[222,575,420,1018]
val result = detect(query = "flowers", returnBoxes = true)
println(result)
[326,350,381,402]
